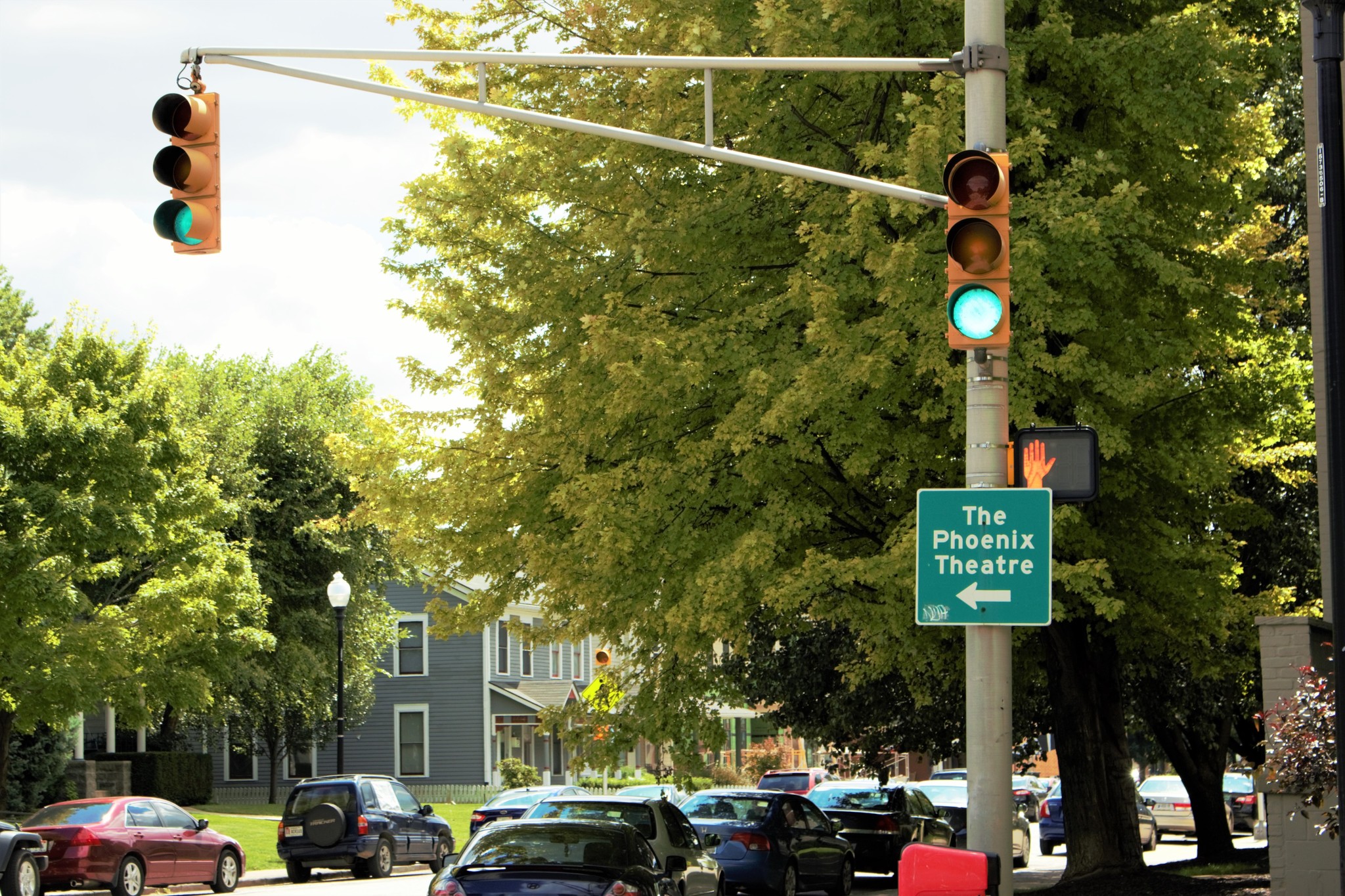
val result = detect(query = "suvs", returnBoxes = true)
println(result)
[0,819,49,896]
[277,773,456,883]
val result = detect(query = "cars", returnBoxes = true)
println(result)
[755,767,840,798]
[674,788,856,896]
[1222,766,1258,836]
[1038,778,1157,855]
[914,766,1053,867]
[13,795,247,896]
[1137,775,1196,841]
[518,794,727,896]
[426,817,683,896]
[615,783,695,806]
[470,785,594,838]
[801,778,956,875]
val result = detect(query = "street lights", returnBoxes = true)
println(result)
[327,569,351,779]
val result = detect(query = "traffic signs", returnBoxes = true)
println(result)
[913,487,1053,628]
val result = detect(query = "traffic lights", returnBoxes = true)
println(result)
[941,141,1014,349]
[151,92,222,255]
[593,723,609,740]
[1013,421,1100,504]
[595,649,611,666]
